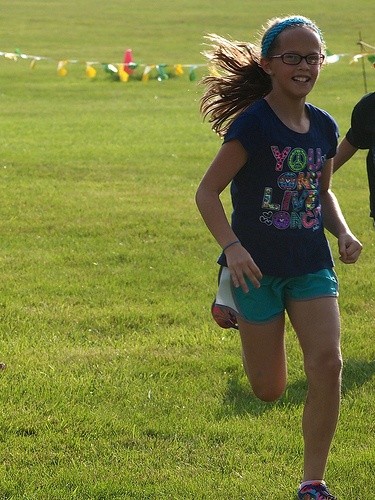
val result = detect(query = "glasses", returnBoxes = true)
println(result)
[269,52,325,66]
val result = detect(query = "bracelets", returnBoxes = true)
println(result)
[222,240,240,252]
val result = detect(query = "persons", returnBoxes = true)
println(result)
[332,92,375,230]
[194,15,362,500]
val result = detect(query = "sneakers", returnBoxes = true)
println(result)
[296,481,338,500]
[212,296,238,330]
